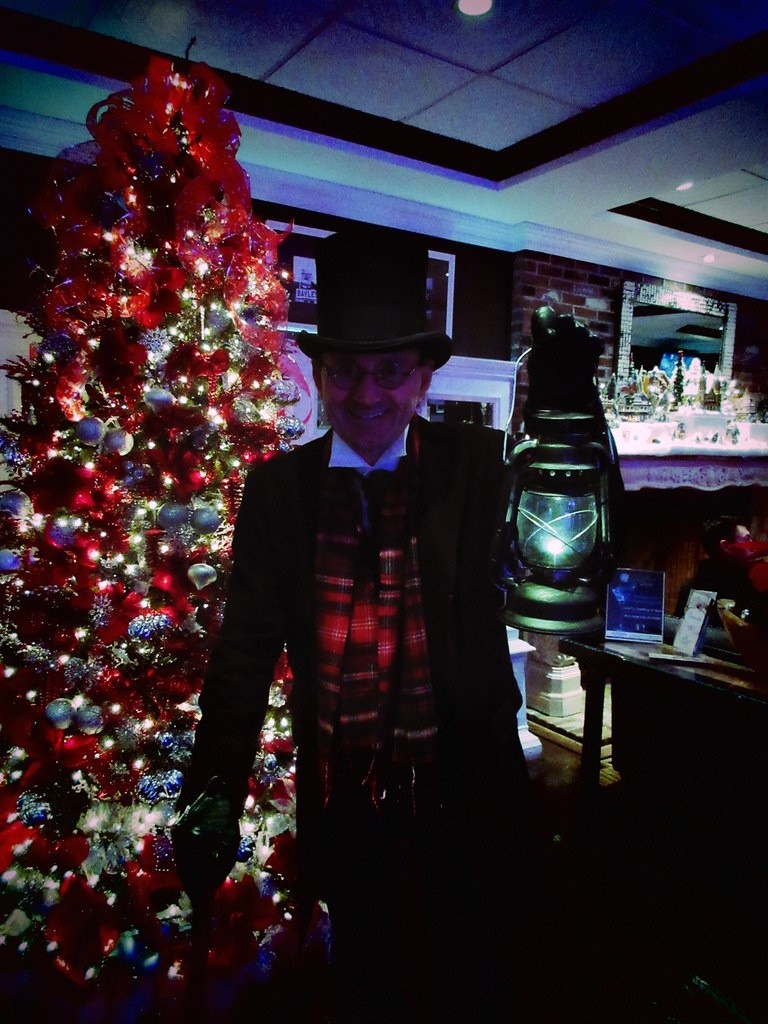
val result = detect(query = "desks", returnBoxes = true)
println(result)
[558,633,768,785]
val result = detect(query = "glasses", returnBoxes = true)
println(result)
[317,361,420,390]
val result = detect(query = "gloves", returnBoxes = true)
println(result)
[171,800,239,916]
[522,305,607,435]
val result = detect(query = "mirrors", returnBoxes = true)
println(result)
[615,280,738,400]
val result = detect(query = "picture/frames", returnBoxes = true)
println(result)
[264,219,456,339]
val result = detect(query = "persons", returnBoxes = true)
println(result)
[167,227,626,1024]
[675,516,768,637]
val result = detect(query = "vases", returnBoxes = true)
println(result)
[717,599,767,682]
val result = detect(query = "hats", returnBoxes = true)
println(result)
[295,228,452,371]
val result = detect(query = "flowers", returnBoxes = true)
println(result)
[728,536,768,593]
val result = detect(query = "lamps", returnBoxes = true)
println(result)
[495,319,628,635]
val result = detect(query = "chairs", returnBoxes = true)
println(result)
[664,615,733,652]
